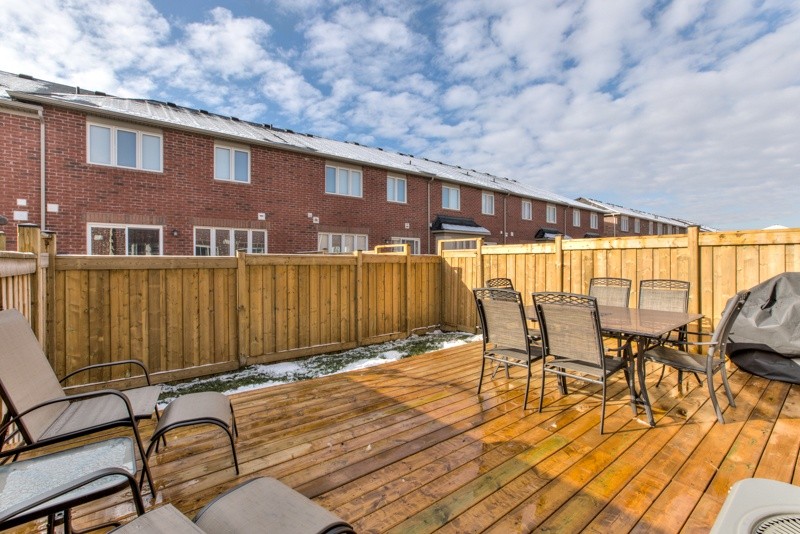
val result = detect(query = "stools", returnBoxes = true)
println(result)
[194,472,358,534]
[133,390,242,488]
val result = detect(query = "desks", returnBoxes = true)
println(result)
[522,304,707,428]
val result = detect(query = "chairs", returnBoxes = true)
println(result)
[0,301,208,534]
[470,276,753,437]
